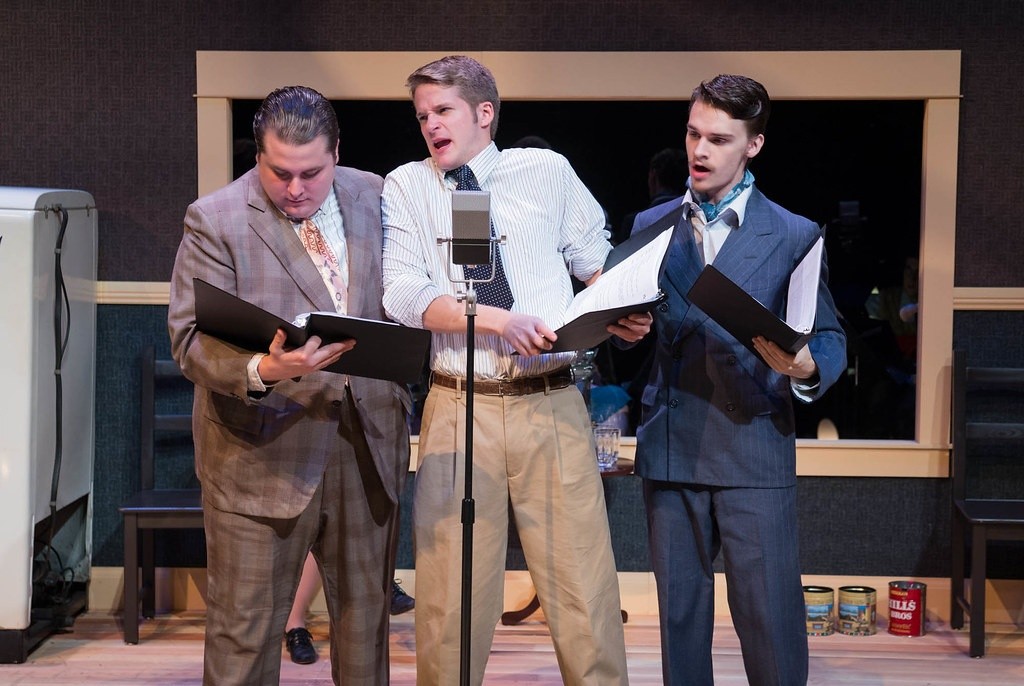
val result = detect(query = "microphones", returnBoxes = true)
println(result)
[450,190,491,265]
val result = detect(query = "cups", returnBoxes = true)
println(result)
[595,429,621,468]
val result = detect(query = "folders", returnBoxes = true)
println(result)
[193,276,429,389]
[509,202,685,355]
[684,222,827,369]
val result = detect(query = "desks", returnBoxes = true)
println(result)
[501,452,636,625]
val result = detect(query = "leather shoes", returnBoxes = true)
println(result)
[390,577,416,617]
[283,627,316,665]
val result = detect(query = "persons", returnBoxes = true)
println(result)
[380,55,654,686]
[167,83,416,686]
[595,75,848,686]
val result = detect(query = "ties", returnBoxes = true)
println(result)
[299,219,337,269]
[444,164,515,312]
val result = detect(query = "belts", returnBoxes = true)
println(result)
[432,370,570,396]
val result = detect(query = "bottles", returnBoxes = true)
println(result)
[571,354,593,422]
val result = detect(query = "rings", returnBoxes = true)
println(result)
[787,365,793,371]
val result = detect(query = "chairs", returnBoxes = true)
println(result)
[950,365,1024,658]
[117,346,206,645]
